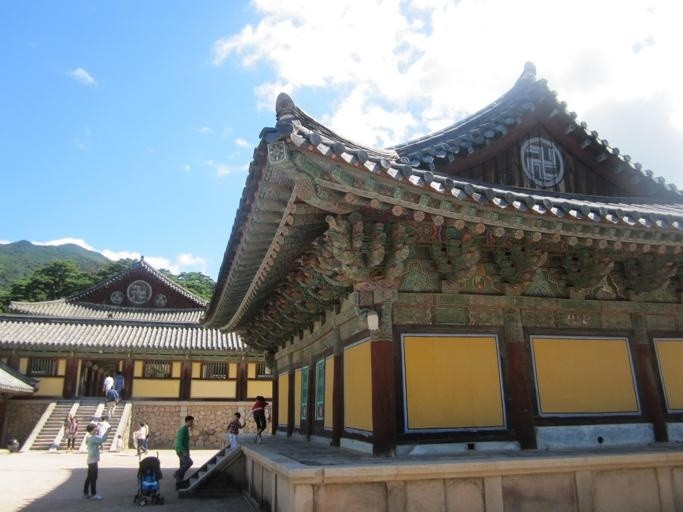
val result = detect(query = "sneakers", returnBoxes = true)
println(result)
[173,471,184,481]
[252,435,263,443]
[83,494,104,501]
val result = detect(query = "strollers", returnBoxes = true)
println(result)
[133,450,164,507]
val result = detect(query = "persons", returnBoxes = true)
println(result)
[101,372,114,398]
[104,386,118,421]
[113,371,125,401]
[134,420,147,457]
[139,420,149,454]
[95,415,110,452]
[244,395,271,444]
[172,416,193,483]
[83,424,111,501]
[64,416,78,449]
[115,434,124,454]
[224,412,246,450]
